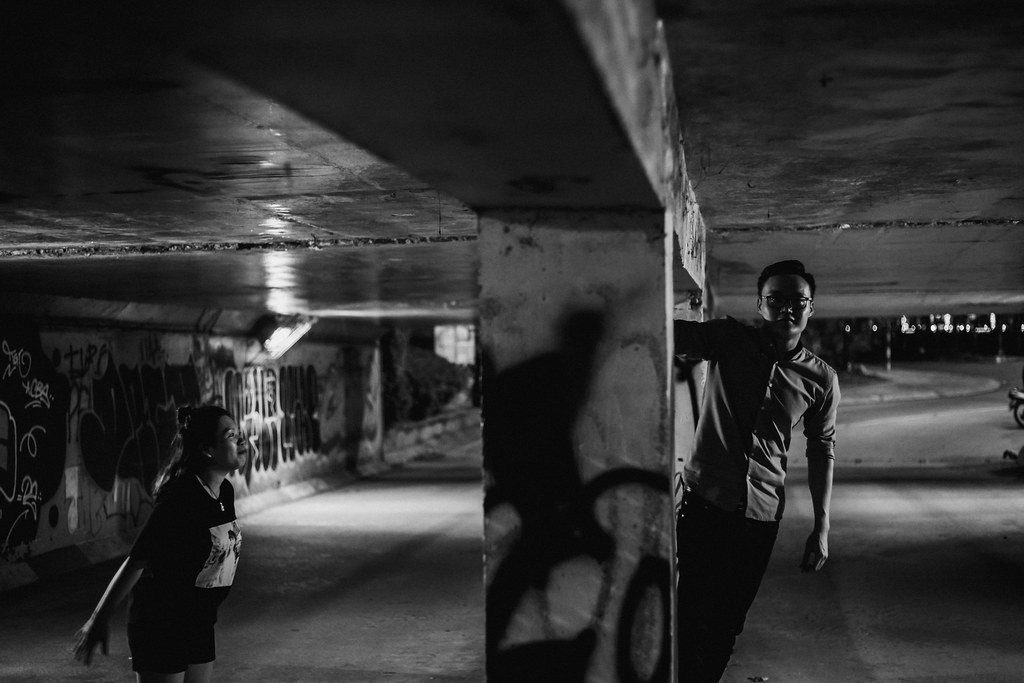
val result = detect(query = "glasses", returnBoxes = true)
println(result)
[762,296,812,309]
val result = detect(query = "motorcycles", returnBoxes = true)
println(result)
[1008,386,1024,429]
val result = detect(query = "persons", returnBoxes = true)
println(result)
[672,258,841,683]
[70,404,249,683]
[205,529,239,588]
